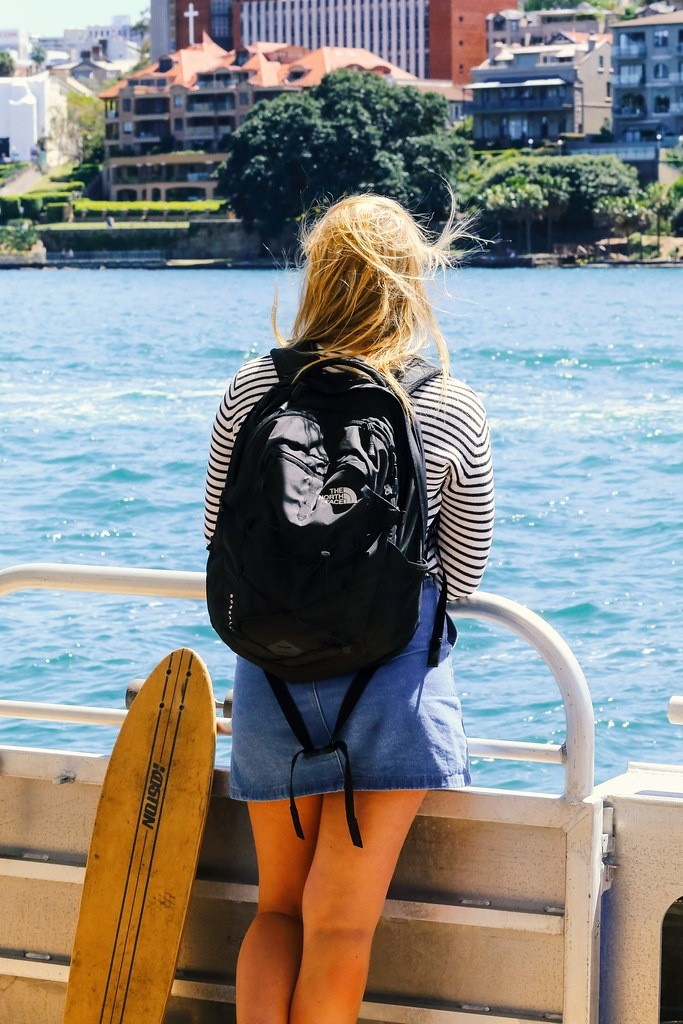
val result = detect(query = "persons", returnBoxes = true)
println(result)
[205,193,493,1024]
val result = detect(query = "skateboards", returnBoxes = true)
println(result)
[59,645,236,1024]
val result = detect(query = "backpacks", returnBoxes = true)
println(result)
[207,347,441,683]
